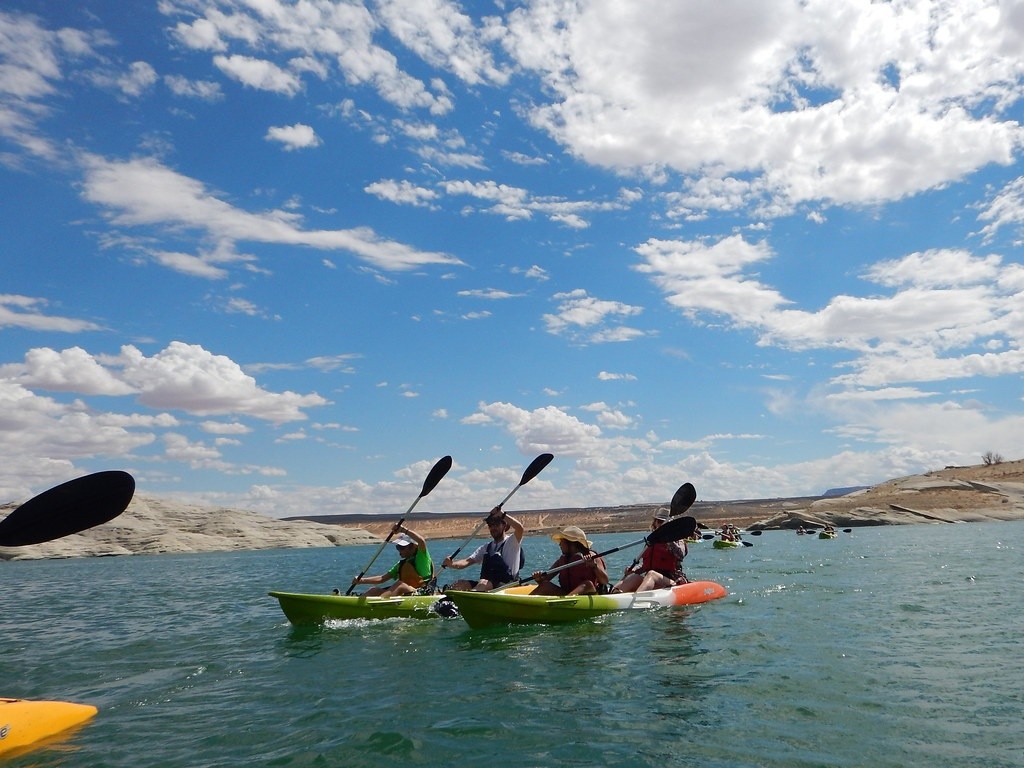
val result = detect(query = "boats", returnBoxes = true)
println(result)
[819,531,837,539]
[713,540,746,548]
[442,580,725,628]
[796,528,807,535]
[268,585,539,627]
[683,538,706,543]
[0,696,97,757]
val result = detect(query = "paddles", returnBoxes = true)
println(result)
[345,455,453,596]
[703,531,762,540]
[434,453,554,578]
[700,524,753,547]
[1,469,136,549]
[807,528,852,534]
[435,516,697,618]
[622,481,698,580]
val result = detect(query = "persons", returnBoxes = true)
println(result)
[824,523,834,534]
[331,523,432,596]
[689,524,701,540]
[610,508,688,593]
[528,525,609,595]
[715,523,742,542]
[443,505,524,592]
[796,525,806,534]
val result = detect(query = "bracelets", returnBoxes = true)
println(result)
[501,512,506,520]
[400,527,408,535]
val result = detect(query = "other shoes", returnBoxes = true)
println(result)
[332,588,340,596]
[598,584,608,595]
[351,592,357,597]
[612,588,623,593]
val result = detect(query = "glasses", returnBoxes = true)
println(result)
[488,521,505,527]
[396,543,412,550]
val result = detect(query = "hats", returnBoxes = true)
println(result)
[484,516,510,532]
[729,524,733,528]
[552,526,593,550]
[652,508,674,522]
[392,533,418,546]
[722,525,728,530]
